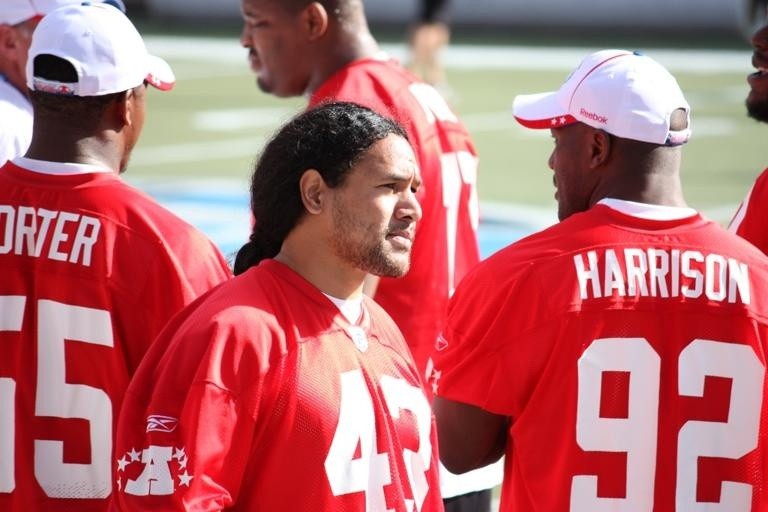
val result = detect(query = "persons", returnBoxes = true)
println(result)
[723,22,768,256]
[0,4,236,511]
[401,7,457,121]
[239,0,482,393]
[0,0,77,171]
[111,100,446,512]
[426,48,768,512]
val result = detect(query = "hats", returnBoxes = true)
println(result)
[512,50,692,147]
[0,0,126,27]
[26,1,176,95]
